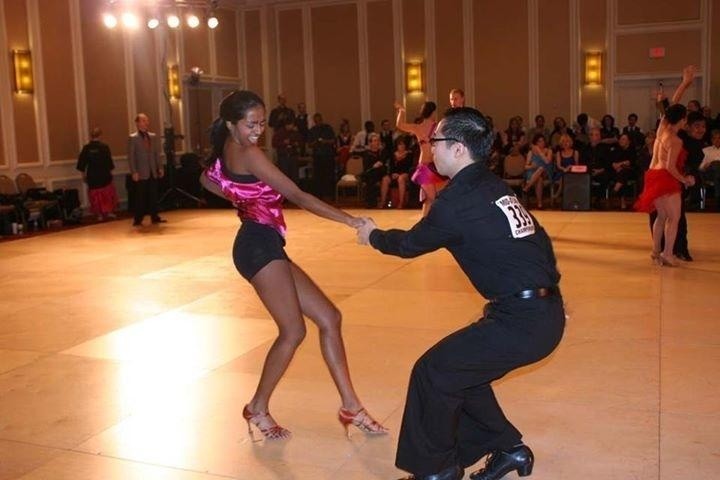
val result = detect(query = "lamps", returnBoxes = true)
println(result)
[12,47,35,94]
[401,54,427,101]
[161,60,182,103]
[97,0,222,35]
[580,47,605,90]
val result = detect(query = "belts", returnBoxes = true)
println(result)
[485,287,561,304]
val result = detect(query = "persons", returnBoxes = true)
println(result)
[76,66,719,267]
[200,88,390,441]
[354,110,565,480]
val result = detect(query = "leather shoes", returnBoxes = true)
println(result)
[397,465,465,480]
[470,445,535,480]
[676,252,692,261]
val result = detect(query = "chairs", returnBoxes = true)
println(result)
[120,142,719,213]
[2,173,82,234]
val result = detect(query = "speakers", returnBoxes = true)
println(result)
[561,173,592,211]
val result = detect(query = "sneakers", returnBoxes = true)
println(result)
[152,220,167,224]
[133,223,144,227]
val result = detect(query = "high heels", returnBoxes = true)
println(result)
[242,404,292,441]
[339,407,391,440]
[650,251,681,268]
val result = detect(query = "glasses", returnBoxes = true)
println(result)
[429,137,460,146]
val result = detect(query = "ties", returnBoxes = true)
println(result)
[143,134,150,152]
